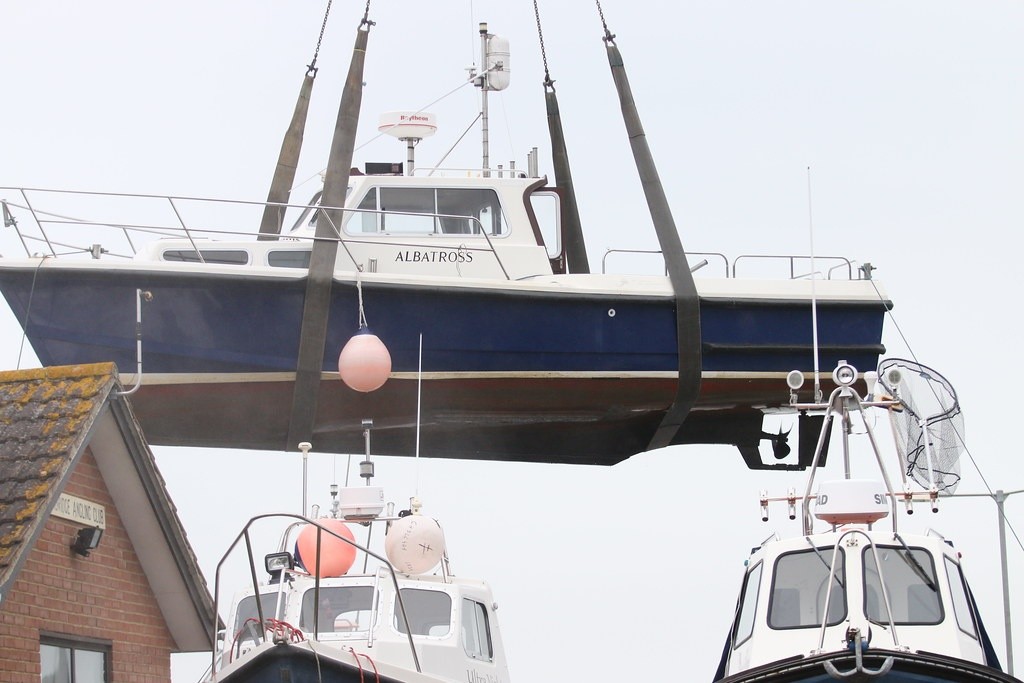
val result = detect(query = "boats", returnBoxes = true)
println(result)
[710,164,1024,683]
[196,332,510,683]
[1,1,893,374]
